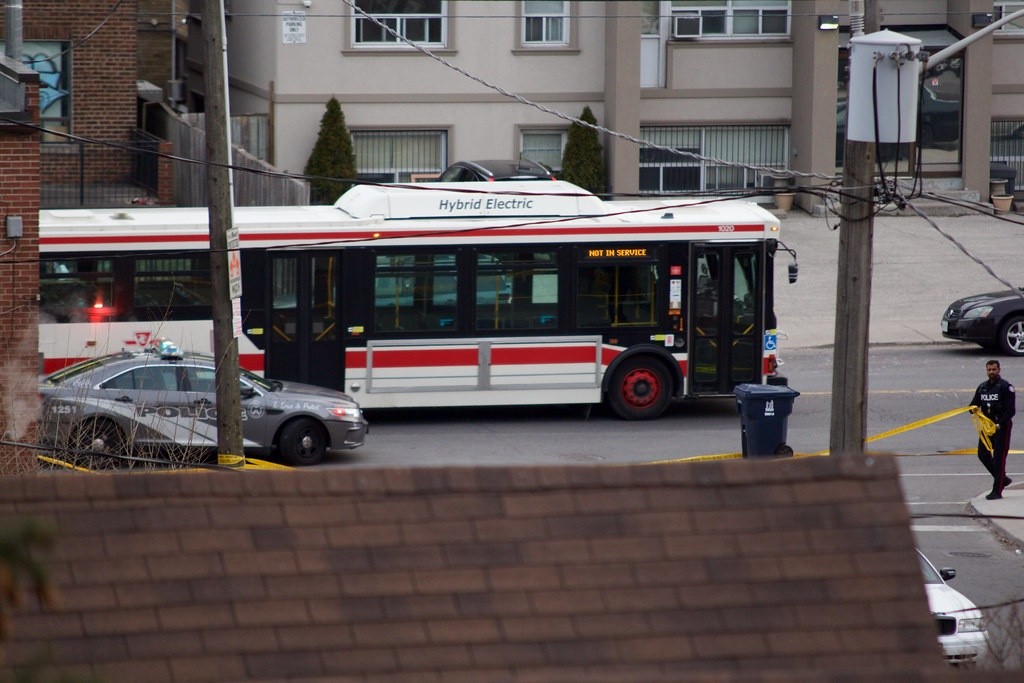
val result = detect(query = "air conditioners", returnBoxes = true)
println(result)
[672,12,703,39]
[758,172,772,192]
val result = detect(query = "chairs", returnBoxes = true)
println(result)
[152,374,165,390]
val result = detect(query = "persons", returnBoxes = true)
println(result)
[968,360,1017,501]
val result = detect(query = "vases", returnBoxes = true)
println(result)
[990,178,1009,194]
[772,173,793,192]
[991,194,1014,215]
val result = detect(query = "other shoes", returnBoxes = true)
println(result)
[986,489,1003,499]
[1003,476,1012,488]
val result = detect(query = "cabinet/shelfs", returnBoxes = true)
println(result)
[775,193,797,211]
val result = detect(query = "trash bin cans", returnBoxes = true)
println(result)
[733,383,800,458]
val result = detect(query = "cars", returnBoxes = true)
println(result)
[33,338,370,469]
[915,548,989,670]
[938,288,1024,357]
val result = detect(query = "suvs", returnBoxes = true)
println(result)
[436,157,556,182]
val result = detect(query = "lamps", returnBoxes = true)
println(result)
[972,14,993,29]
[819,14,839,31]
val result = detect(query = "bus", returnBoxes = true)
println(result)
[37,182,801,423]
